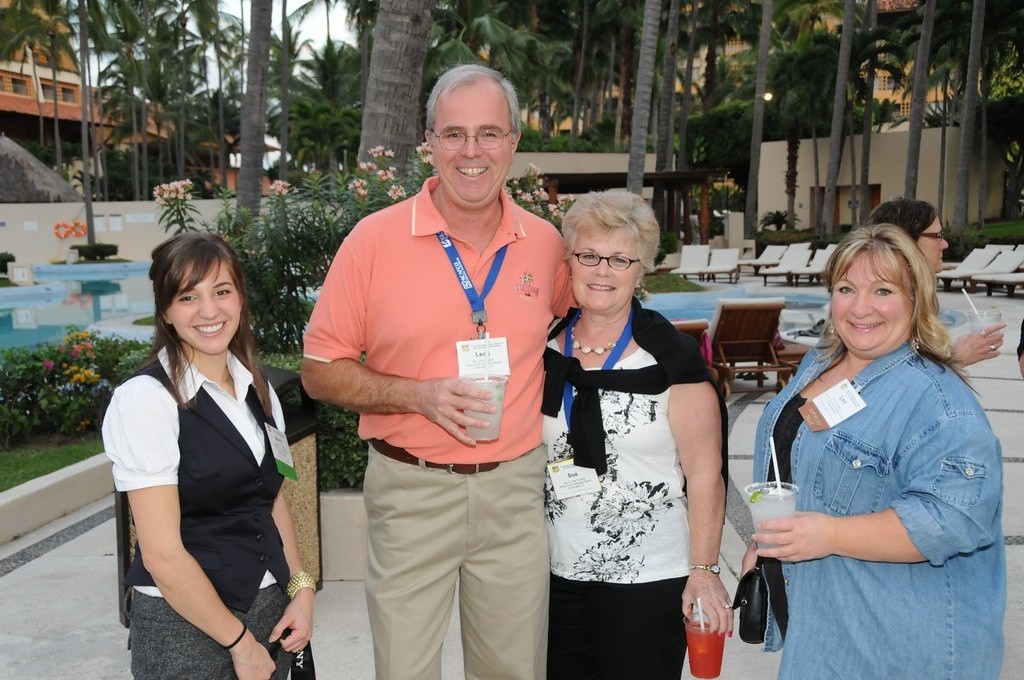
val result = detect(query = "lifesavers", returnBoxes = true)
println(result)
[65,293,92,306]
[54,220,87,238]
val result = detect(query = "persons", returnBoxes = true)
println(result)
[739,222,1007,680]
[868,196,1007,371]
[299,66,578,680]
[1018,319,1024,379]
[102,234,317,680]
[538,188,735,680]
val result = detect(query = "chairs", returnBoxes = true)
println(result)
[812,249,825,264]
[969,273,1024,298]
[760,249,811,285]
[1015,244,1024,252]
[709,296,791,391]
[787,242,811,250]
[956,252,1024,293]
[738,245,788,274]
[781,326,821,346]
[671,318,709,346]
[936,248,1001,292]
[699,248,740,284]
[792,244,838,284]
[670,244,710,282]
[983,243,1015,252]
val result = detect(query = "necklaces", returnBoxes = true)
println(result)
[571,324,618,354]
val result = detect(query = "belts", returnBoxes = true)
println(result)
[368,437,500,474]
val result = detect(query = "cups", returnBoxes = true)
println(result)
[746,482,799,549]
[683,611,724,679]
[968,309,1002,338]
[461,374,508,440]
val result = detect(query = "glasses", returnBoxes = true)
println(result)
[920,230,943,240]
[431,127,514,151]
[573,252,640,270]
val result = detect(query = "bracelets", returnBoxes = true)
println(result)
[287,571,316,599]
[222,625,247,649]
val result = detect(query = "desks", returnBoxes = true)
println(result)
[777,342,813,390]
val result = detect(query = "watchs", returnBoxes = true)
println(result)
[688,563,720,574]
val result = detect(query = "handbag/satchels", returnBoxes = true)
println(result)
[733,569,767,643]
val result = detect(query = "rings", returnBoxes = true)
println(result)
[980,330,987,336]
[990,345,994,351]
[725,603,732,609]
